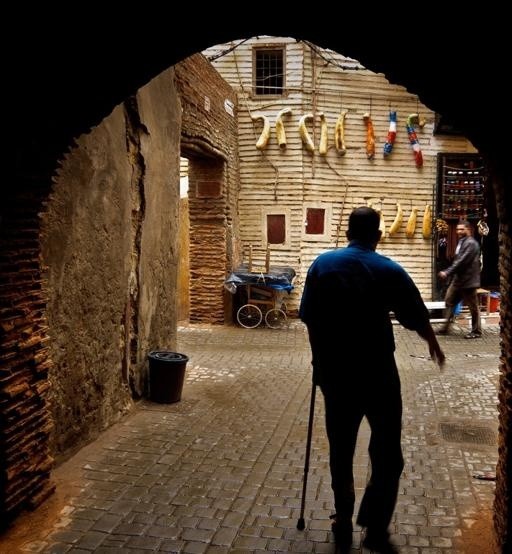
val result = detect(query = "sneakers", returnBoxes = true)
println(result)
[364,525,403,553]
[331,521,354,535]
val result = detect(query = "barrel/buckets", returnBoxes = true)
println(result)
[147,350,189,404]
[481,292,500,313]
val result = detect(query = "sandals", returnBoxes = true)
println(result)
[465,330,481,338]
[434,328,448,335]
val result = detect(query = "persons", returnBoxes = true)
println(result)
[300,205,445,550]
[433,222,484,340]
